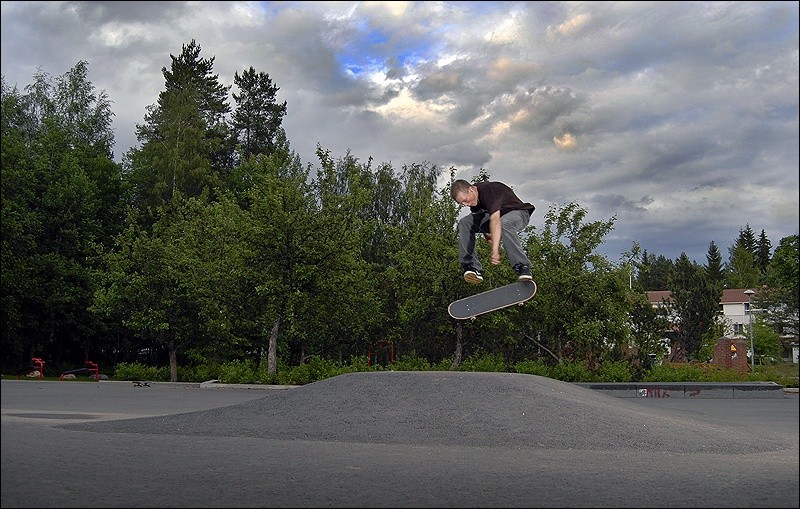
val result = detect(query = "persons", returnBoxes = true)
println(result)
[450,178,536,283]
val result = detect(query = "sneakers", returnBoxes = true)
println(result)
[464,268,484,284]
[516,263,532,282]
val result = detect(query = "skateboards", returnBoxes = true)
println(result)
[447,277,538,320]
